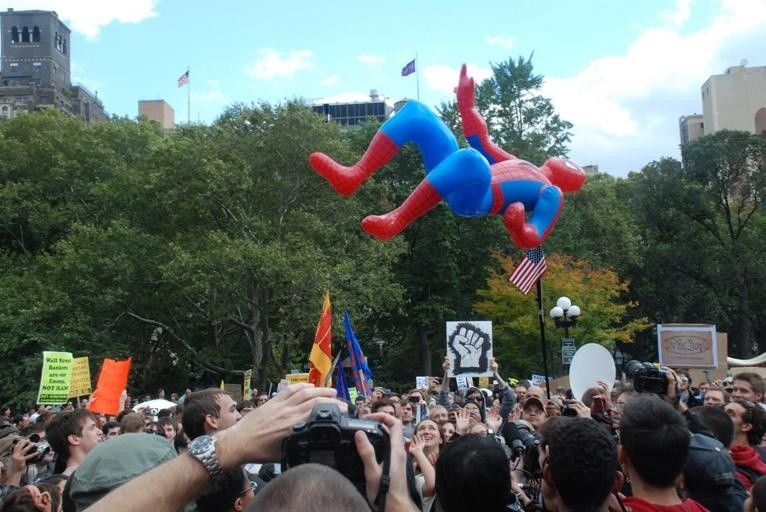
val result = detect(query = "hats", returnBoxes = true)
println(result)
[682,433,736,488]
[0,432,40,459]
[523,398,545,412]
[62,432,198,512]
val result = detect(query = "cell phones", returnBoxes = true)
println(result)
[408,396,420,402]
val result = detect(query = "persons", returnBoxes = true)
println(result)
[2,361,766,510]
[307,61,586,201]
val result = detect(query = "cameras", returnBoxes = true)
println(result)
[13,434,50,464]
[144,408,158,416]
[560,400,577,416]
[624,360,668,394]
[283,405,390,512]
[502,421,545,479]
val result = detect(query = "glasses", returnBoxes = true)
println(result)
[466,396,483,402]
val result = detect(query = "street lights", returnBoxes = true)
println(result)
[549,295,582,374]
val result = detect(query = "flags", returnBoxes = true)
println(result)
[176,70,189,88]
[506,242,548,296]
[400,58,415,77]
[342,310,373,399]
[307,288,334,389]
